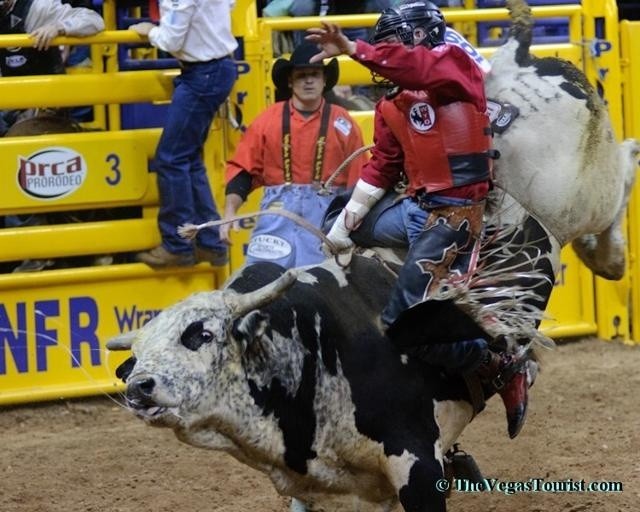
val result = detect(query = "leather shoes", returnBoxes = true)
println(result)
[489,351,539,440]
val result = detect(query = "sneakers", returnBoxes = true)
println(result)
[13,257,54,273]
[137,241,229,267]
[89,252,112,266]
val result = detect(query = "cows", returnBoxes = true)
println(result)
[105,0,640,512]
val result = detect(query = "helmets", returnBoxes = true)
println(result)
[373,1,447,50]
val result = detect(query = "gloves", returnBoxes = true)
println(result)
[320,208,355,267]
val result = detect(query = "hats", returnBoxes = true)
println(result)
[271,44,340,96]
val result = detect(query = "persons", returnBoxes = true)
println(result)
[1,1,474,270]
[299,1,546,440]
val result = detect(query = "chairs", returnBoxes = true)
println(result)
[0,0,606,228]
